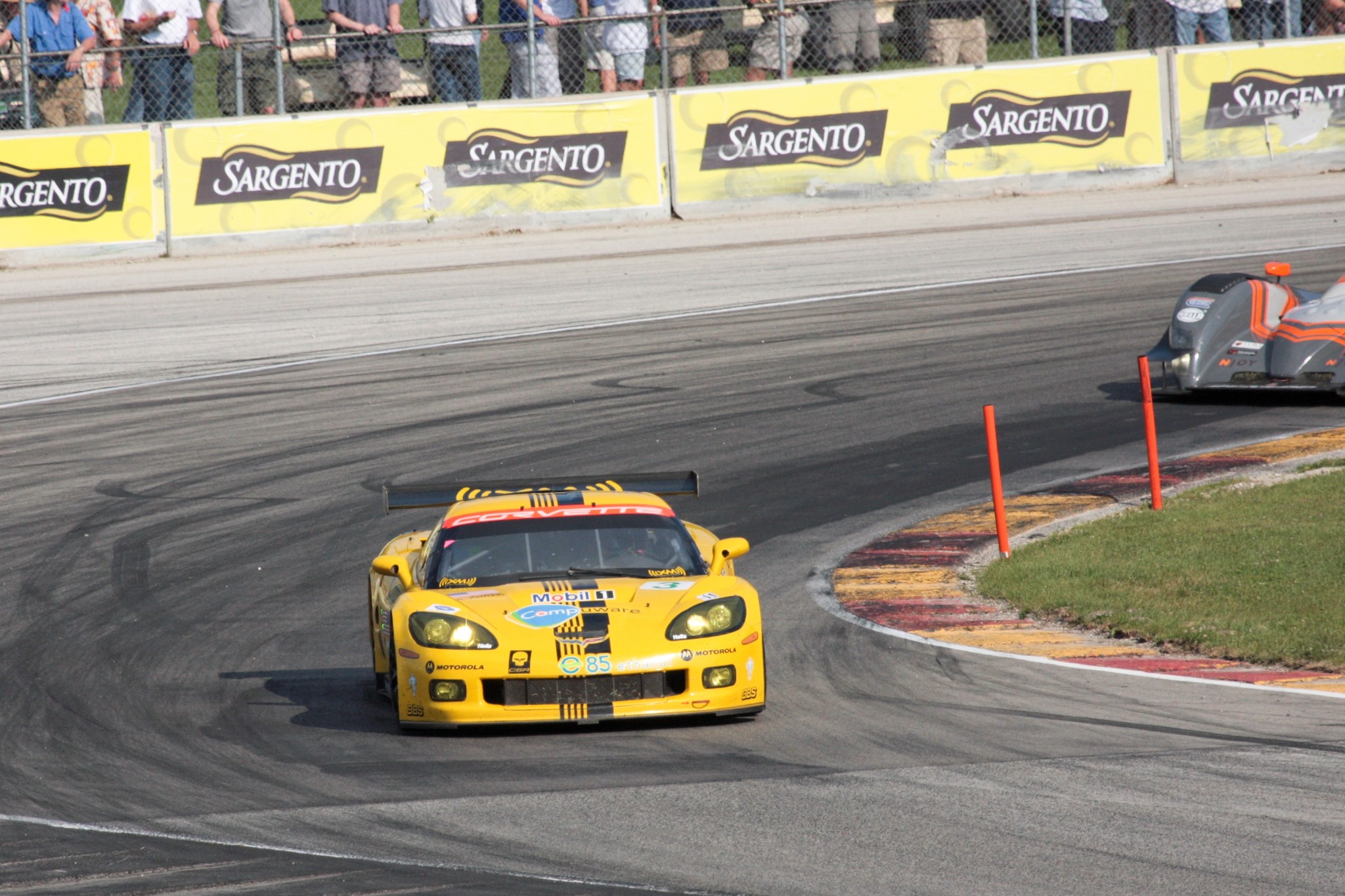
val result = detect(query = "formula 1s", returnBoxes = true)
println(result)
[1140,261,1345,407]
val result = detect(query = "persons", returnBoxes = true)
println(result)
[321,0,404,110]
[206,0,303,117]
[418,0,489,103]
[497,0,989,99]
[1049,0,1345,57]
[0,0,204,129]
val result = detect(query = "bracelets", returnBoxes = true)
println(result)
[651,30,660,37]
[109,65,120,71]
[286,24,295,28]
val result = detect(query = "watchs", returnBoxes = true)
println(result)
[189,29,198,35]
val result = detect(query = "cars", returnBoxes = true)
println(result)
[369,469,765,735]
